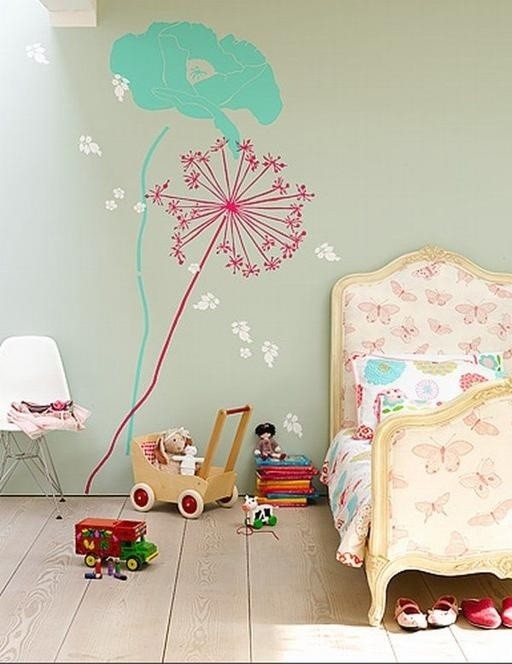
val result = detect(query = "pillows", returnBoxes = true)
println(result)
[348,347,503,446]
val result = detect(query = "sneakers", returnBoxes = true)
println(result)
[394,595,512,630]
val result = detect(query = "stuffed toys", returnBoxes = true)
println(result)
[152,425,194,471]
[171,444,203,477]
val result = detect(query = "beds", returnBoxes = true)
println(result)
[320,245,512,627]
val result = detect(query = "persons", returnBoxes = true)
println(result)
[96,555,102,573]
[107,557,114,575]
[114,556,121,572]
[254,422,287,462]
[113,572,127,580]
[84,571,103,578]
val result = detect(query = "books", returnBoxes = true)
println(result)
[254,453,319,509]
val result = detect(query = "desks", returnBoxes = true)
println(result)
[0,425,68,520]
